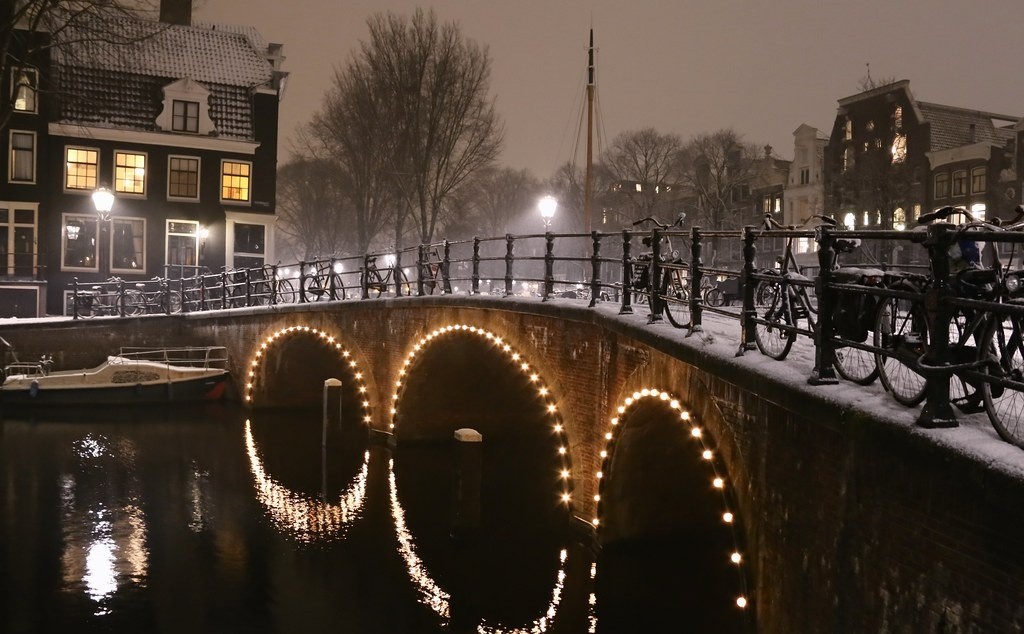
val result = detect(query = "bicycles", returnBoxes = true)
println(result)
[303,256,345,302]
[872,205,1024,448]
[671,266,780,307]
[216,265,256,308]
[421,238,453,295]
[182,267,212,312]
[114,276,184,316]
[632,213,693,329]
[360,250,410,299]
[752,212,891,385]
[76,276,138,319]
[255,258,295,305]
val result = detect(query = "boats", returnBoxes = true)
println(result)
[0,338,232,408]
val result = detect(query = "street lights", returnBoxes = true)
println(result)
[92,180,116,288]
[536,195,559,293]
